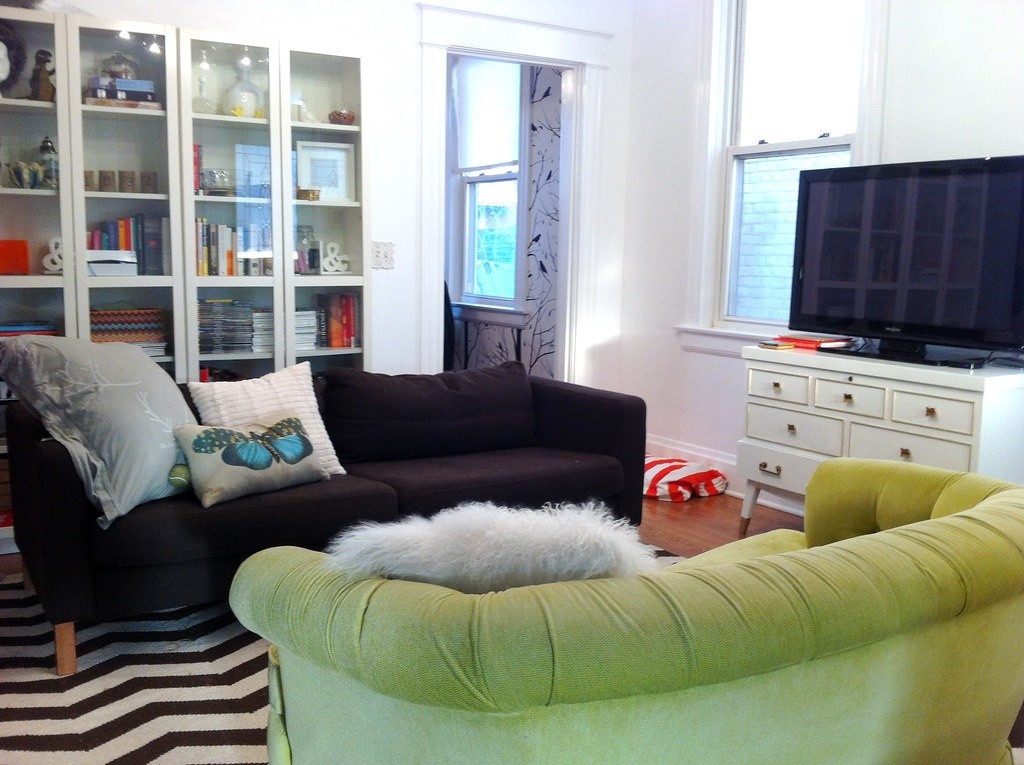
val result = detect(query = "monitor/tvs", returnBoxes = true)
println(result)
[788,154,1024,368]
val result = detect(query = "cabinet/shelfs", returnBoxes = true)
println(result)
[0,7,368,454]
[737,347,1024,538]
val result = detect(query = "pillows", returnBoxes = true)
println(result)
[0,330,198,531]
[324,494,641,590]
[174,408,326,510]
[186,354,350,480]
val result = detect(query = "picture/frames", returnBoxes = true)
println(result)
[295,141,355,200]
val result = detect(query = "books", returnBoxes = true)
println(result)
[295,291,361,350]
[195,215,273,276]
[192,143,204,194]
[84,76,164,110]
[197,297,274,354]
[0,320,64,337]
[758,333,857,350]
[87,212,172,275]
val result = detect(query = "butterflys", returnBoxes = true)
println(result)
[191,415,313,471]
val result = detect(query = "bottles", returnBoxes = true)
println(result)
[221,66,264,119]
[100,50,140,79]
[36,136,59,191]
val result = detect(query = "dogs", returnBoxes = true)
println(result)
[321,497,660,601]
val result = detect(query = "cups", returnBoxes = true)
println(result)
[118,170,137,193]
[84,170,95,191]
[99,169,116,192]
[141,171,158,194]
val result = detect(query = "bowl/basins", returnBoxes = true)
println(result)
[298,189,320,201]
[328,110,354,125]
[5,160,45,189]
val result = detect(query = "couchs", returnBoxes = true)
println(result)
[7,359,652,684]
[227,453,1024,765]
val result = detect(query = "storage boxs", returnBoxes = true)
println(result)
[113,78,156,93]
[114,90,156,102]
[0,239,29,274]
[91,77,114,90]
[85,250,139,276]
[92,89,116,99]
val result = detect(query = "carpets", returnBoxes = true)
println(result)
[0,533,692,765]
